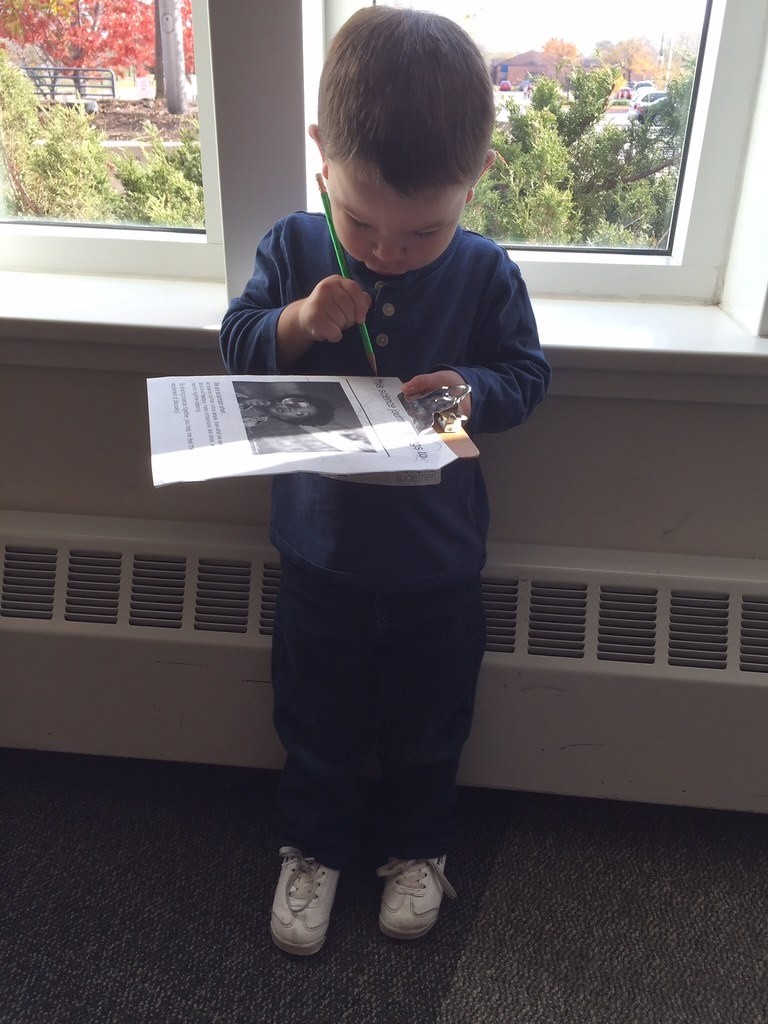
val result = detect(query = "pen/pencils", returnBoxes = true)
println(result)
[315,172,380,379]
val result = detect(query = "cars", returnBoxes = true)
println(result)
[614,82,679,126]
[500,81,511,91]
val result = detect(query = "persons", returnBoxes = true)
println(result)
[234,392,336,440]
[219,4,552,956]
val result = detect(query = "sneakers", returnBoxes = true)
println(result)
[376,851,456,940]
[270,845,341,956]
